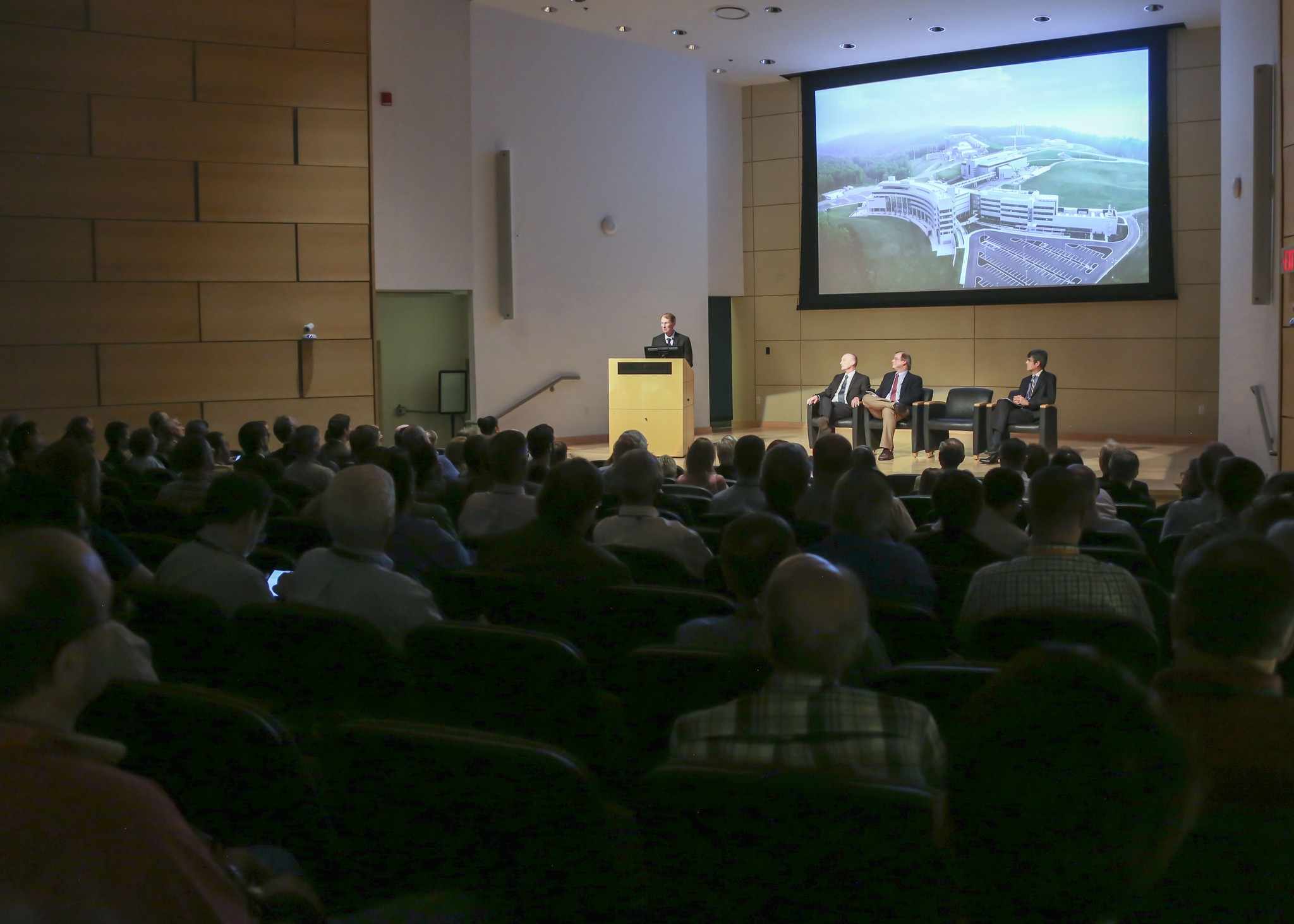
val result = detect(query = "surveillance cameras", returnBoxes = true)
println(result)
[303,323,314,333]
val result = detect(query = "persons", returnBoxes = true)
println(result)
[0,403,1294,924]
[977,348,1058,465]
[651,312,694,369]
[805,352,871,443]
[862,350,924,462]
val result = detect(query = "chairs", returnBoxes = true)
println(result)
[985,390,1057,462]
[864,387,934,457]
[924,387,993,460]
[80,474,1293,924]
[808,389,875,453]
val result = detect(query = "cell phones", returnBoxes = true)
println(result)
[265,567,295,599]
[235,455,243,461]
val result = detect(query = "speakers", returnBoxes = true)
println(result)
[1252,63,1276,305]
[496,150,513,320]
[1251,382,1279,458]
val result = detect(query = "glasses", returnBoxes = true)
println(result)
[1028,359,1039,363]
[893,357,901,361]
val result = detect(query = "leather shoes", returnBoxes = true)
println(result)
[979,452,999,463]
[878,448,894,460]
[984,443,1000,454]
[893,402,907,415]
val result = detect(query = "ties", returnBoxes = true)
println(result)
[1026,375,1036,400]
[890,373,899,401]
[667,338,671,346]
[838,375,848,404]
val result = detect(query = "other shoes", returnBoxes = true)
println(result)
[817,417,832,433]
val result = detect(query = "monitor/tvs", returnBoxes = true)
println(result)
[644,346,683,358]
